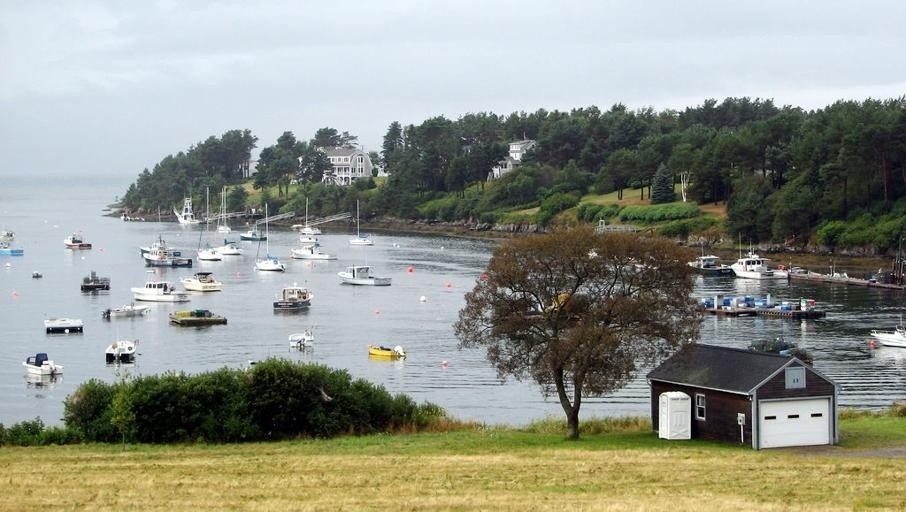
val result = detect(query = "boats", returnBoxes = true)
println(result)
[62,230,93,251]
[746,336,815,368]
[97,301,151,319]
[0,229,24,258]
[102,336,140,363]
[132,237,409,365]
[681,255,733,277]
[79,268,109,291]
[790,235,905,290]
[725,246,790,282]
[21,353,63,375]
[118,213,145,222]
[686,294,829,319]
[873,346,906,365]
[44,317,84,335]
[869,323,906,350]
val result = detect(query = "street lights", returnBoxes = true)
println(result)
[788,254,793,273]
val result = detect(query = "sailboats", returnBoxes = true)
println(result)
[171,186,377,269]
